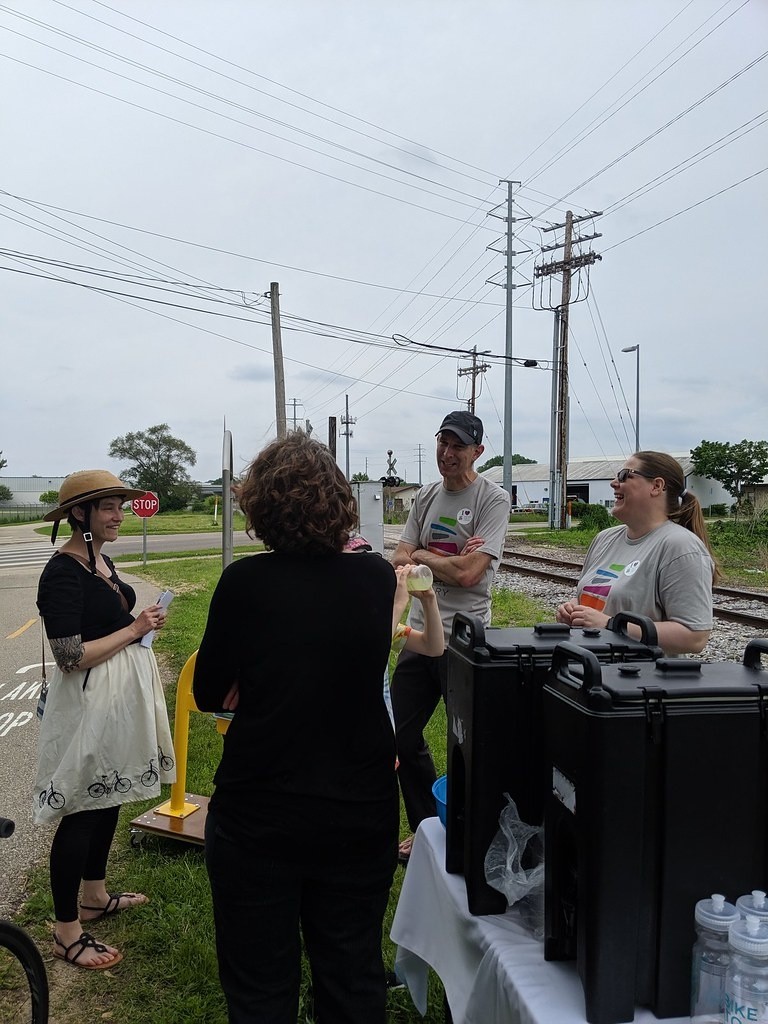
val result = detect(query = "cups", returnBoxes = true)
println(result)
[405,564,434,592]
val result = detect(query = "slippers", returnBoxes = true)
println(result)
[398,835,415,863]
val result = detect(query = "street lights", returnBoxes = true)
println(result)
[621,342,641,453]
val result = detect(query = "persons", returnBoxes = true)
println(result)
[30,470,177,970]
[556,450,722,658]
[390,411,510,862]
[193,430,445,1024]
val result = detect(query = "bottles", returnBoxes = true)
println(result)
[688,890,768,1024]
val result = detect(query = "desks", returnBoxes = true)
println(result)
[388,817,725,1024]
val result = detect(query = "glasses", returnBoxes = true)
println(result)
[616,468,666,491]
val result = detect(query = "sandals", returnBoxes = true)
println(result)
[79,889,149,923]
[52,930,123,970]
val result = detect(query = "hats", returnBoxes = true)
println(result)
[43,470,147,522]
[435,410,483,446]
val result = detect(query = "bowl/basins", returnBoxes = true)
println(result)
[432,774,446,830]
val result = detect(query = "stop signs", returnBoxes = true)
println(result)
[131,491,159,518]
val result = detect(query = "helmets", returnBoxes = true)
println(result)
[342,531,373,552]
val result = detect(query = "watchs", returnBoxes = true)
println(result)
[606,616,615,630]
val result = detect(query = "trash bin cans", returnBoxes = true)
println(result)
[541,636,768,1024]
[441,609,665,917]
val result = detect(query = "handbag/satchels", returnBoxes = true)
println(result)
[37,687,49,720]
